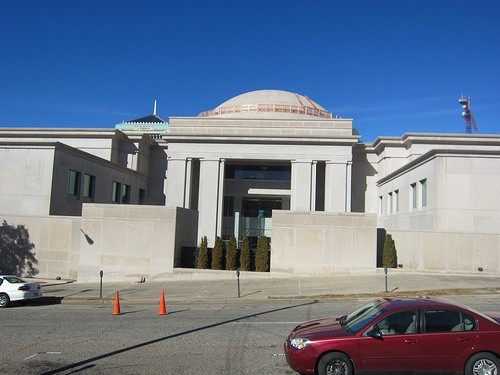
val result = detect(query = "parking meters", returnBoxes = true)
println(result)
[384,266,388,292]
[237,269,240,297]
[99,270,103,298]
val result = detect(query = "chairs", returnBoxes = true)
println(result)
[402,314,416,334]
[378,321,398,336]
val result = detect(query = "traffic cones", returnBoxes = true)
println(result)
[111,290,121,315]
[155,289,168,315]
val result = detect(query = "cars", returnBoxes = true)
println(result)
[283,296,500,375]
[0,275,42,307]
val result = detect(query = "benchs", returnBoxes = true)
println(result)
[443,311,473,331]
